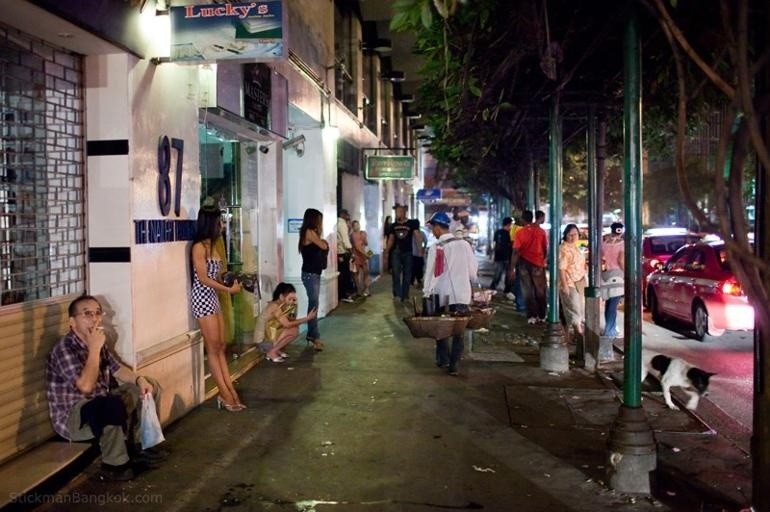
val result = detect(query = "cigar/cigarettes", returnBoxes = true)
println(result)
[95,326,104,329]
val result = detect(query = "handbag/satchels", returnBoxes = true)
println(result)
[575,277,587,293]
[222,272,256,292]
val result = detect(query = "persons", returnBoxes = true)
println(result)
[419,212,480,377]
[337,209,354,303]
[252,282,318,363]
[350,221,374,297]
[190,205,247,413]
[600,222,625,337]
[44,296,168,482]
[558,224,589,345]
[298,208,329,351]
[382,203,423,302]
[383,209,547,302]
[511,211,549,326]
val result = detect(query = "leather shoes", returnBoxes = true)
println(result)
[134,443,167,464]
[264,350,289,362]
[99,465,133,480]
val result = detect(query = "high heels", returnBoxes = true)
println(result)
[217,394,247,411]
[306,337,325,351]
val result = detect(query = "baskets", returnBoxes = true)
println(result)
[403,314,471,340]
[468,307,495,329]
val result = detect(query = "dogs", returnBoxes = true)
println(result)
[622,348,718,412]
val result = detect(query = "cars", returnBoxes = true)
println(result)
[647,242,756,340]
[642,229,701,302]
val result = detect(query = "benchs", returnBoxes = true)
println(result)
[0,296,92,512]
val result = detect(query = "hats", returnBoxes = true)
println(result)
[425,212,450,225]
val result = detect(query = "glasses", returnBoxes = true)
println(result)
[76,310,105,317]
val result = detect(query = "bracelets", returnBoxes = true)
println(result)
[228,287,230,293]
[135,376,141,386]
